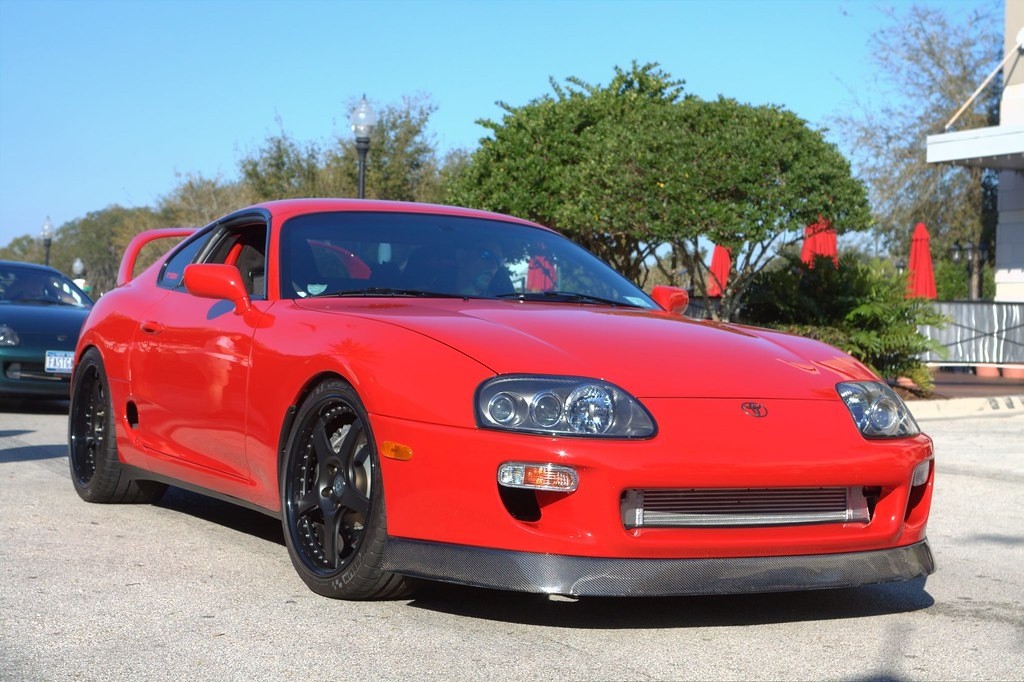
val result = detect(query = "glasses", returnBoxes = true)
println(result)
[471,246,507,268]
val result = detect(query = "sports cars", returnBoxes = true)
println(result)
[67,197,936,604]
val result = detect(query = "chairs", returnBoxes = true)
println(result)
[402,245,515,296]
[225,226,323,297]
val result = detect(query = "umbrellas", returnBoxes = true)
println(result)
[526,243,558,292]
[799,195,839,272]
[706,241,732,296]
[903,222,937,300]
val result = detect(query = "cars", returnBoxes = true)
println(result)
[0,260,100,400]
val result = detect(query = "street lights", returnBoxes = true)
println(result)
[950,231,998,301]
[41,214,56,265]
[348,92,377,199]
[71,257,85,288]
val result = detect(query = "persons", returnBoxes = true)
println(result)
[452,234,507,295]
